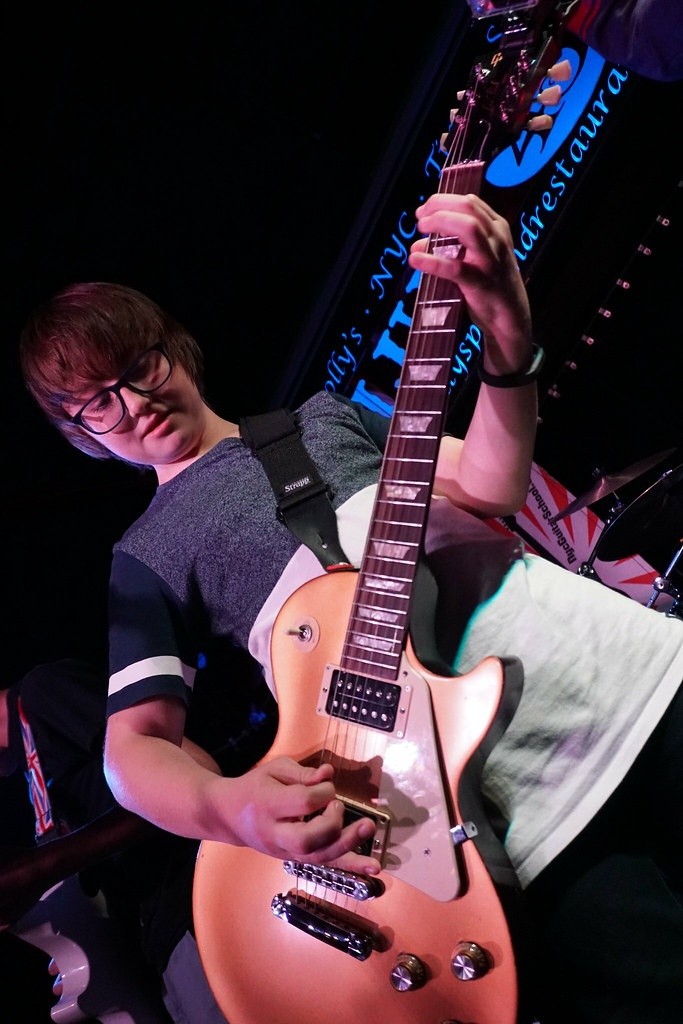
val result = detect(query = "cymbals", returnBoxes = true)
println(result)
[547,445,681,527]
[594,462,683,563]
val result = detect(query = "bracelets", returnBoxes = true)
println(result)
[476,342,542,386]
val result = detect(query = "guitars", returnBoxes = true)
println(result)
[4,777,170,1024]
[188,0,604,1023]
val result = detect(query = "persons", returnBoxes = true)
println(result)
[23,193,682,1024]
[0,661,195,1024]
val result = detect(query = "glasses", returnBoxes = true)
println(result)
[72,337,173,436]
